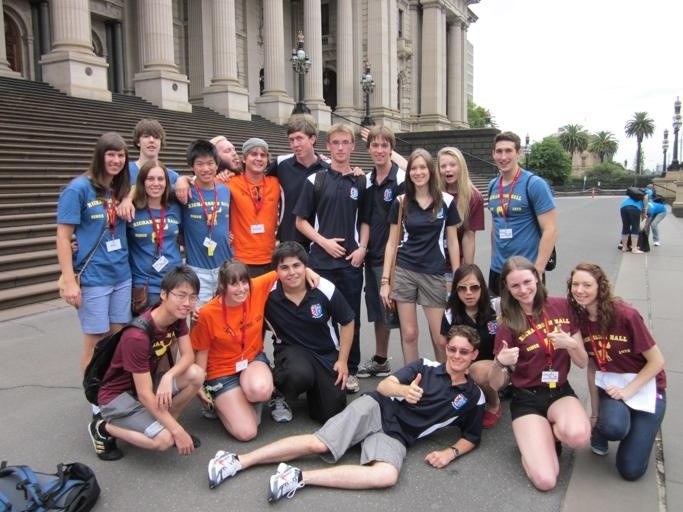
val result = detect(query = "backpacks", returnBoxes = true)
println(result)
[82,316,154,406]
[1,460,100,512]
[488,175,556,271]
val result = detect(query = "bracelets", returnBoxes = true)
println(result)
[449,444,460,459]
[493,358,505,370]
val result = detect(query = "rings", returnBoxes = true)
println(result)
[516,354,519,358]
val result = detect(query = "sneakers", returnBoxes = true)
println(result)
[356,357,392,377]
[201,404,217,420]
[619,241,659,254]
[207,450,241,489]
[480,403,502,429]
[267,390,294,423]
[88,418,124,460]
[590,428,609,456]
[346,374,360,393]
[267,462,305,503]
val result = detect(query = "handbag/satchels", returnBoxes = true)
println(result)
[384,284,397,314]
[627,186,645,202]
[131,285,148,313]
[58,273,82,299]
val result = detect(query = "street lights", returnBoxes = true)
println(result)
[668,96,681,171]
[660,129,669,177]
[288,30,312,115]
[359,64,375,126]
[523,134,531,170]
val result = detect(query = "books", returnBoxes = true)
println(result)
[593,367,657,415]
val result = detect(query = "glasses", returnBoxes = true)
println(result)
[167,290,200,305]
[447,344,474,355]
[455,284,481,292]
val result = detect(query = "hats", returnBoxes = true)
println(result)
[242,138,269,155]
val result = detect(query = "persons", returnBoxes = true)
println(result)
[639,199,669,247]
[438,265,512,429]
[54,115,485,415]
[485,131,558,297]
[189,258,321,442]
[206,324,485,506]
[187,241,356,425]
[492,254,592,493]
[564,261,668,480]
[86,265,206,461]
[619,187,653,255]
